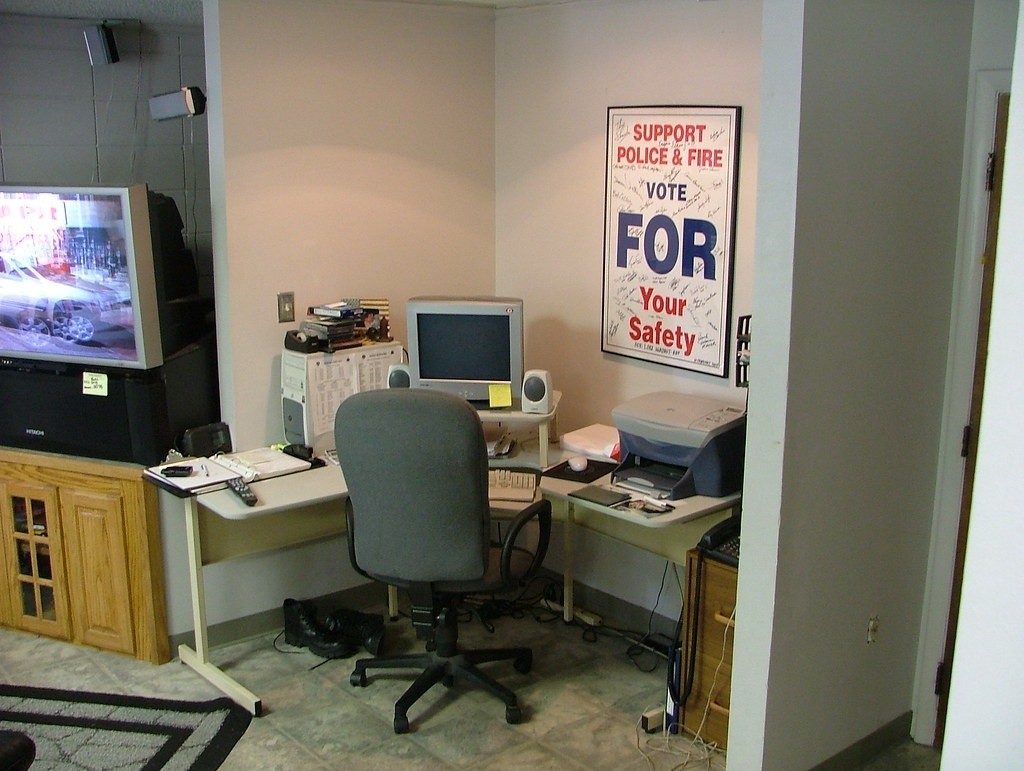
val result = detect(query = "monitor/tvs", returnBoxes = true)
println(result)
[0,183,203,377]
[408,296,525,411]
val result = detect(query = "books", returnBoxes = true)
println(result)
[301,302,363,349]
[145,446,325,499]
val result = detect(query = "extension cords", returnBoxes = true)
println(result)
[540,596,602,626]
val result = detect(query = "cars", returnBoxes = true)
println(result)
[0,252,121,346]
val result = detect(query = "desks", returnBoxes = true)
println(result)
[179,447,744,716]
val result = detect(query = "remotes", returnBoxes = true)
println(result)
[227,477,258,506]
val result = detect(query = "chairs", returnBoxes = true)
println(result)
[335,390,554,732]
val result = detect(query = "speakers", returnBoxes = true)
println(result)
[83,26,120,65]
[149,86,206,120]
[521,369,554,414]
[386,365,411,389]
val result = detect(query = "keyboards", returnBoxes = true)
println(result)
[488,469,536,502]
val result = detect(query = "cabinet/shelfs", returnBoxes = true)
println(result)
[677,550,740,752]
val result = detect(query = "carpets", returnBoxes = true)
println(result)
[0,685,251,771]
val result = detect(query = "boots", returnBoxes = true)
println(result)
[282,598,348,657]
[333,606,385,655]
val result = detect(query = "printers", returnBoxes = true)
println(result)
[610,391,747,501]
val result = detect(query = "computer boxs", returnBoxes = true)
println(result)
[281,339,404,455]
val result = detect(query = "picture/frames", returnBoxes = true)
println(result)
[600,105,742,378]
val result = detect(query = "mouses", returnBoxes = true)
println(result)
[568,455,587,471]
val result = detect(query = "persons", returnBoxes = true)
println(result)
[365,312,380,329]
